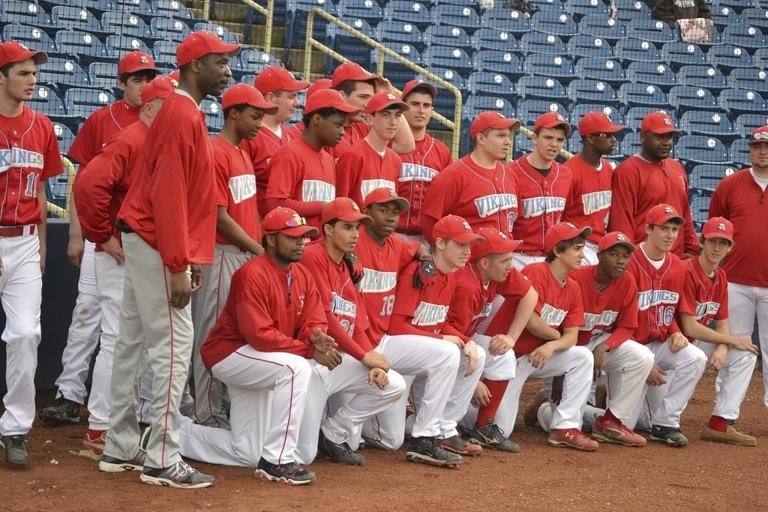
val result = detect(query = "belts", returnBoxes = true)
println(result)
[0,224,35,236]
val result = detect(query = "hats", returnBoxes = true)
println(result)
[176,31,241,66]
[432,214,485,243]
[599,231,634,252]
[321,197,374,225]
[263,206,319,237]
[471,227,524,259]
[403,80,435,98]
[303,89,359,116]
[254,65,310,96]
[141,77,175,104]
[579,111,624,136]
[118,51,162,76]
[0,42,48,68]
[306,79,332,97]
[533,112,570,137]
[703,217,734,244]
[367,90,409,114]
[640,112,676,135]
[645,204,685,225]
[747,126,768,143]
[222,83,279,115]
[333,63,380,87]
[545,222,592,252]
[471,112,520,139]
[365,188,410,211]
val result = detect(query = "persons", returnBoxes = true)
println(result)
[36,30,767,491]
[0,40,65,469]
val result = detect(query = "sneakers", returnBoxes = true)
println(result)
[406,435,464,468]
[650,424,688,446]
[701,425,756,446]
[79,430,106,462]
[98,449,146,473]
[592,413,647,446]
[254,456,315,485]
[524,389,552,424]
[318,428,365,465]
[40,390,80,423]
[0,433,28,466]
[433,434,481,455]
[470,418,520,453]
[139,461,214,489]
[546,428,598,450]
